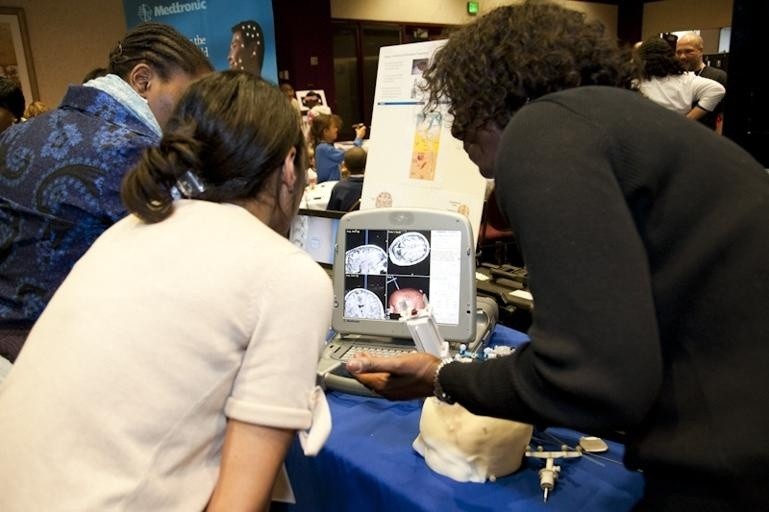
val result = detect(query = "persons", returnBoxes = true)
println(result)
[634,37,727,124]
[670,32,728,132]
[2,19,216,369]
[345,2,768,511]
[1,69,335,511]
[276,77,367,207]
[219,21,273,79]
[1,79,25,134]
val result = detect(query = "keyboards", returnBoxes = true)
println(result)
[318,337,470,399]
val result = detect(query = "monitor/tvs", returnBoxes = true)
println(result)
[333,207,477,343]
[286,208,345,271]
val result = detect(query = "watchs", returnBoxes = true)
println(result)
[433,357,456,405]
